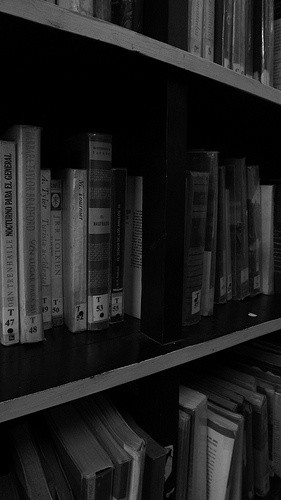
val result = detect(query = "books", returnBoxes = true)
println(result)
[0,340,281,500]
[45,0,281,92]
[0,114,281,345]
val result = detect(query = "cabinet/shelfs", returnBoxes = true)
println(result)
[0,0,281,423]
